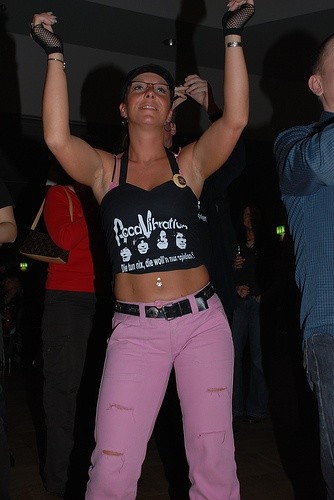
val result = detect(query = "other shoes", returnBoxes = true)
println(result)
[45,484,86,500]
[10,452,15,467]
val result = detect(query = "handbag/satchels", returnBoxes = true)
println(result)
[20,185,73,264]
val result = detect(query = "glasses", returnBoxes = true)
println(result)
[129,81,170,96]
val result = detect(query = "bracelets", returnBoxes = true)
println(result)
[47,58,66,68]
[225,41,242,48]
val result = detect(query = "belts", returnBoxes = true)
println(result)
[114,282,216,321]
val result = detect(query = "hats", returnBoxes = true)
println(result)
[122,63,176,99]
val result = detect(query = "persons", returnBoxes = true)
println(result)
[0,174,18,249]
[164,74,252,335]
[41,156,114,496]
[0,262,27,370]
[30,0,255,500]
[275,32,334,500]
[230,205,271,435]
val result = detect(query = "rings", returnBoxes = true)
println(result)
[30,21,34,25]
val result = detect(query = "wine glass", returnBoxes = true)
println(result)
[233,242,244,269]
